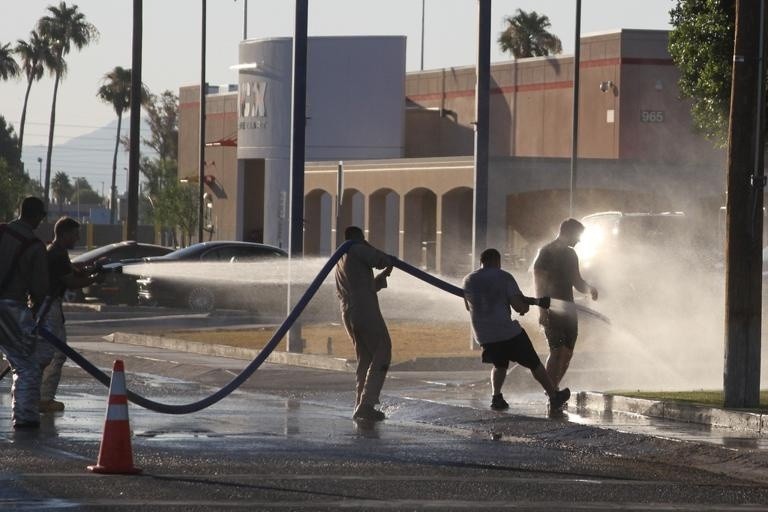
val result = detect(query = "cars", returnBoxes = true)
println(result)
[63,240,178,301]
[133,236,301,310]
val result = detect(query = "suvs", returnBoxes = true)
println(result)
[526,210,714,279]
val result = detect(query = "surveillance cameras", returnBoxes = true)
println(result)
[599,81,608,93]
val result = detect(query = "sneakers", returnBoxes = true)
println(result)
[352,404,387,421]
[38,398,66,414]
[548,387,570,411]
[489,392,510,409]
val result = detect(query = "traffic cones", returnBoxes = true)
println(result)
[84,356,147,484]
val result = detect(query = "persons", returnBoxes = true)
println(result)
[334,224,397,420]
[26,215,110,414]
[461,247,571,410]
[532,215,599,410]
[0,194,51,431]
